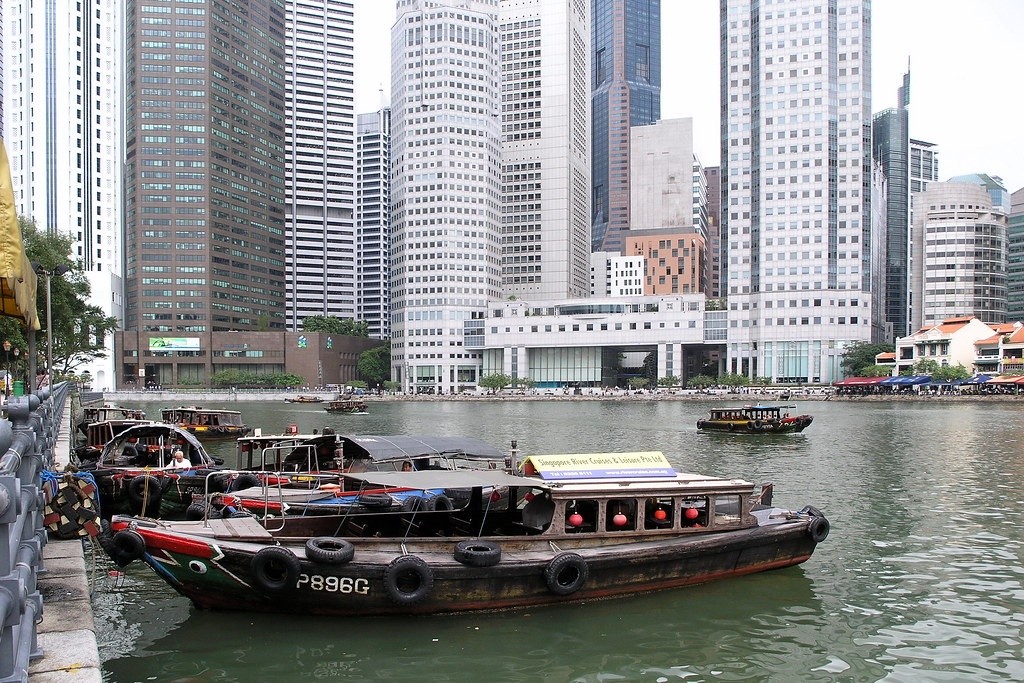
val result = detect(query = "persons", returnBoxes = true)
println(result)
[837,386,915,395]
[166,451,192,468]
[401,461,412,472]
[917,386,1024,395]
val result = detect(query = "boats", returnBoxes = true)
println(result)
[696,401,815,435]
[324,395,368,413]
[41,407,829,616]
[284,395,323,403]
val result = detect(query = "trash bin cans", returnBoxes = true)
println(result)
[12,381,25,397]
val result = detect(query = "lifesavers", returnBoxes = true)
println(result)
[401,496,430,513]
[746,421,754,430]
[233,473,263,491]
[358,493,392,508]
[697,419,703,429]
[185,500,220,520]
[801,504,824,518]
[111,529,145,560]
[428,494,454,512]
[727,422,734,431]
[305,537,355,564]
[544,551,590,595]
[383,555,435,605]
[249,545,301,592]
[754,420,763,429]
[207,425,253,434]
[453,539,503,567]
[808,515,830,542]
[128,475,162,504]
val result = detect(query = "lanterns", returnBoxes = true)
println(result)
[613,515,627,525]
[176,440,184,444]
[569,514,583,526]
[686,509,698,519]
[490,492,500,501]
[130,438,136,442]
[525,493,534,501]
[655,510,666,519]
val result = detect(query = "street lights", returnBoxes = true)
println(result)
[31,261,71,397]
[3,341,11,400]
[14,347,19,381]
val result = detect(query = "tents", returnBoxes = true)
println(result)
[833,375,932,386]
[931,375,1024,385]
